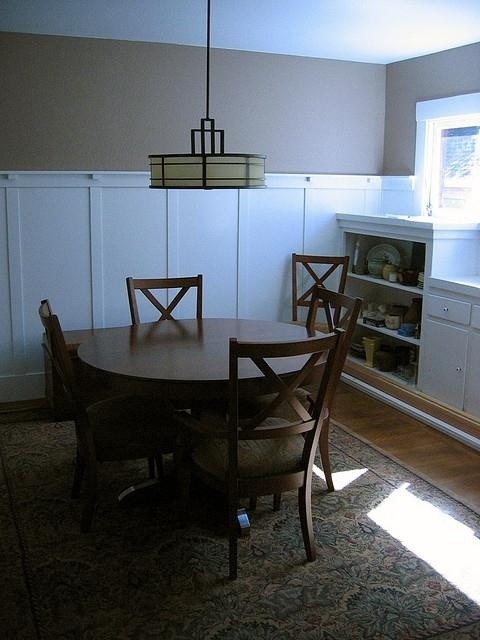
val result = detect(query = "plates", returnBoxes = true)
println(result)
[416,271,424,288]
[366,244,401,275]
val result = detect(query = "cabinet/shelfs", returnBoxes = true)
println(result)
[337,221,426,391]
[419,289,478,418]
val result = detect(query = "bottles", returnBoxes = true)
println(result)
[382,260,397,282]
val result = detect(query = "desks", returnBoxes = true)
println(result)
[78,319,330,505]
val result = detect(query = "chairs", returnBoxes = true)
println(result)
[179,328,346,579]
[37,295,163,497]
[247,284,362,513]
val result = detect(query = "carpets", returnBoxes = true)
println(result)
[0,417,479,640]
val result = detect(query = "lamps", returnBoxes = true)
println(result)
[148,0,269,190]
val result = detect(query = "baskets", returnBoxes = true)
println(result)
[417,272,425,290]
